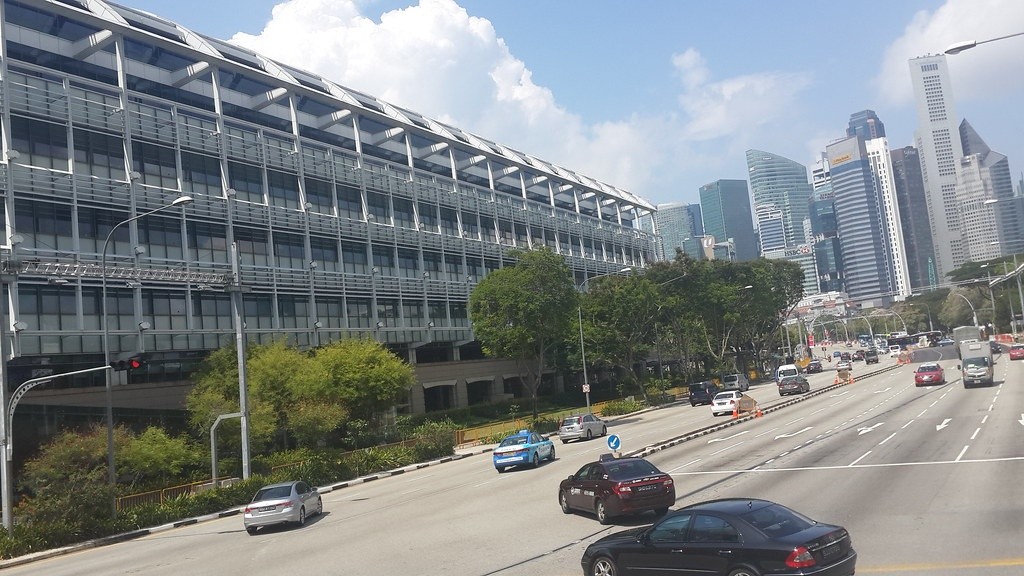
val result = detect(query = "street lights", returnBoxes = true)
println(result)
[101,196,196,517]
[910,304,933,331]
[578,267,632,411]
[981,263,1024,315]
[713,286,753,384]
[653,272,691,403]
[949,294,979,327]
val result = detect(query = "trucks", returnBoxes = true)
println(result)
[952,326,998,388]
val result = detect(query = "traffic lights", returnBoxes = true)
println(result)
[109,353,145,372]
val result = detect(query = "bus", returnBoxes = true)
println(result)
[857,331,944,348]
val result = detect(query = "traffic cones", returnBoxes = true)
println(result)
[849,374,856,384]
[732,406,737,418]
[756,406,762,417]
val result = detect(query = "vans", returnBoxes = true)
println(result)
[689,381,720,406]
[723,374,750,391]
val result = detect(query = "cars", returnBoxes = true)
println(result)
[913,363,945,386]
[990,343,1001,354]
[580,498,856,576]
[774,359,823,395]
[557,456,677,525]
[937,339,955,347]
[244,481,323,536]
[493,430,556,473]
[710,391,749,416]
[1008,343,1024,360]
[834,341,901,371]
[559,413,607,444]
